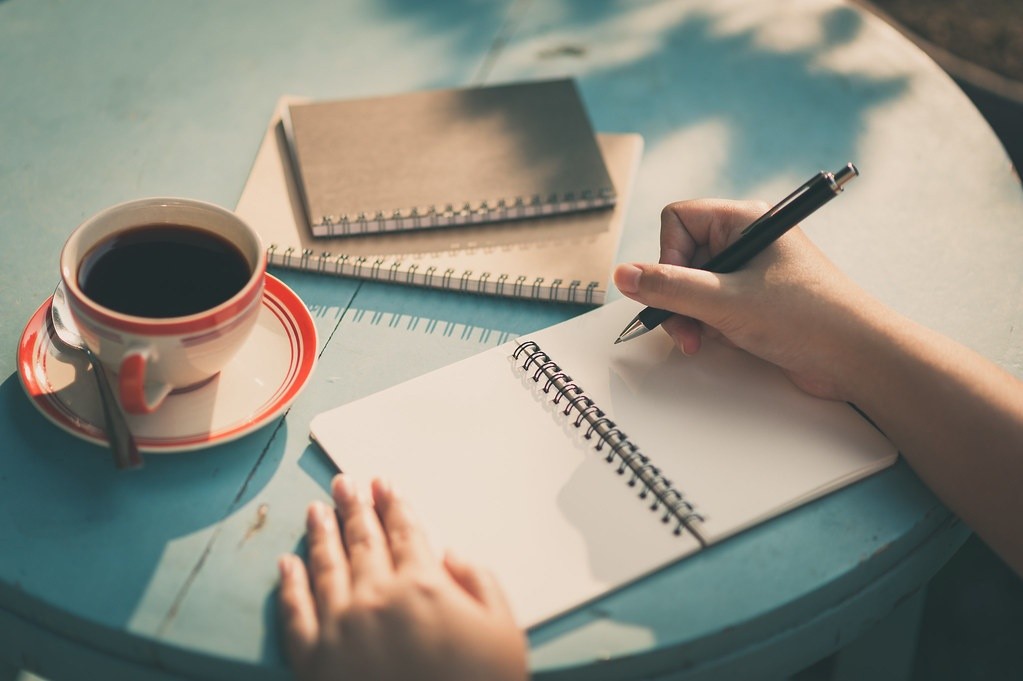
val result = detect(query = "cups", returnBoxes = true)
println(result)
[59,196,268,414]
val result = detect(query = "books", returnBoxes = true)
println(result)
[234,77,643,307]
[309,298,899,631]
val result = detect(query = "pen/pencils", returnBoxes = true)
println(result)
[613,160,859,348]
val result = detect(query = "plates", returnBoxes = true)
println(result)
[16,270,319,452]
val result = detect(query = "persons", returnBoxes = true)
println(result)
[278,196,1023,681]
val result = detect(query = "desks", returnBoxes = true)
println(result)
[0,0,1023,681]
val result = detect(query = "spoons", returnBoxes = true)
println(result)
[51,277,144,472]
